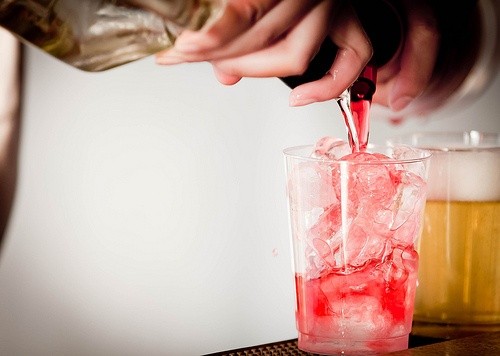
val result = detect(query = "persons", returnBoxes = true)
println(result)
[2,0,500,129]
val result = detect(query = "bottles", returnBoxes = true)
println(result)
[349,0,441,101]
[0,0,335,93]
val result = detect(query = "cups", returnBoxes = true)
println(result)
[410,129,500,325]
[282,142,434,355]
